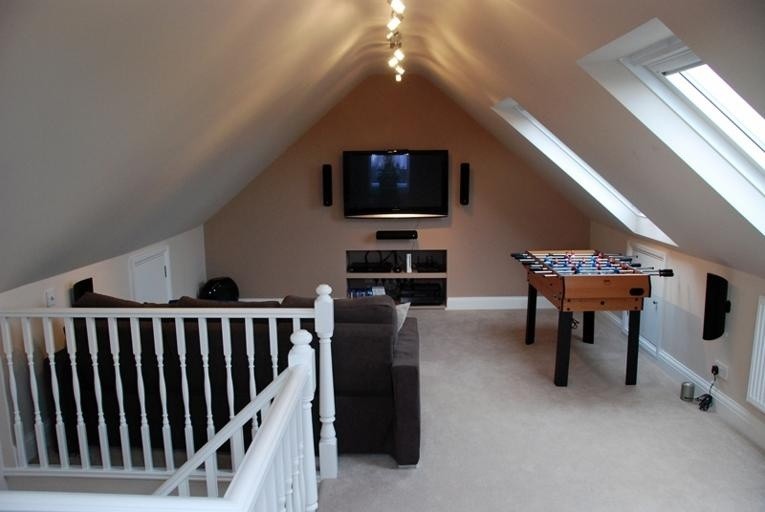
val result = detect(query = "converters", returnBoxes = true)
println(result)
[711,365,718,375]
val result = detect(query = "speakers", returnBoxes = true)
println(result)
[460,163,469,205]
[322,164,332,206]
[703,272,731,340]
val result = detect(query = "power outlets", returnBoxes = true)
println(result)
[712,360,728,380]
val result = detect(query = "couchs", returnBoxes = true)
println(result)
[43,292,421,469]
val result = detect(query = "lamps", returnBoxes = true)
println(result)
[386,0,406,82]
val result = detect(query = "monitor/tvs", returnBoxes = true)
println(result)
[343,150,448,219]
